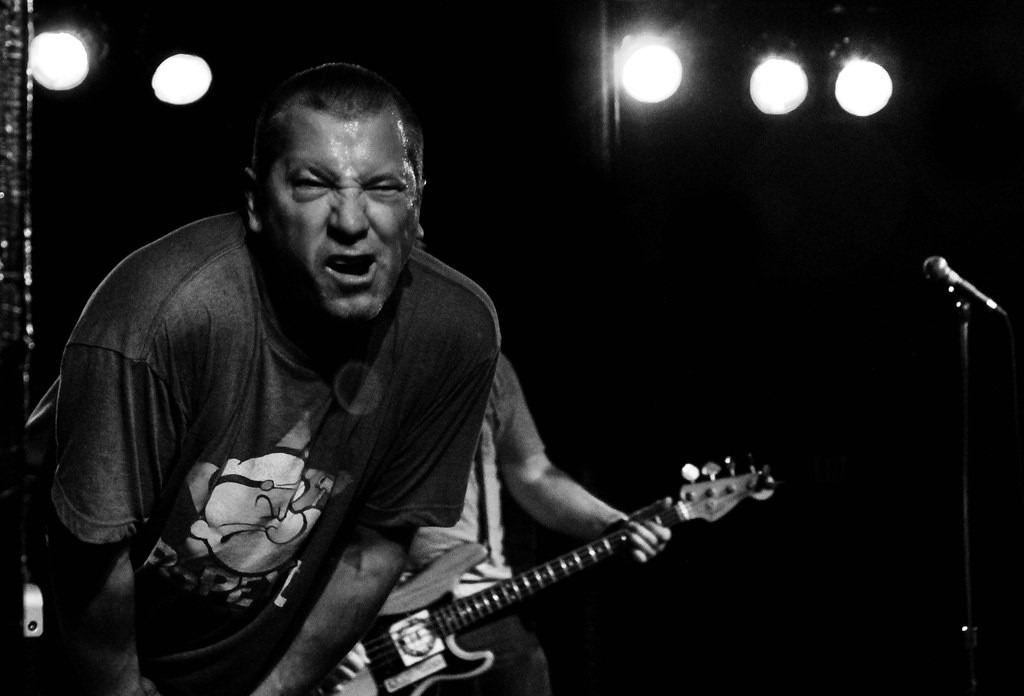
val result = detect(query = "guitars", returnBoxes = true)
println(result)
[303,455,776,696]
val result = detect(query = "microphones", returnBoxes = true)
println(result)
[923,254,1006,319]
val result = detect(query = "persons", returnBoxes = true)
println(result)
[308,352,673,695]
[18,57,498,695]
